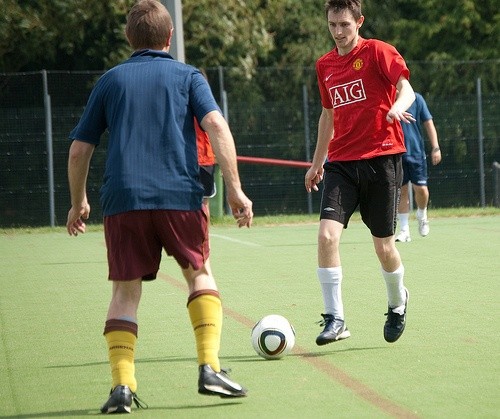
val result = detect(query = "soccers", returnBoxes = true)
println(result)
[250,314,297,360]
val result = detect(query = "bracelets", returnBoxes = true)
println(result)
[432,147,439,151]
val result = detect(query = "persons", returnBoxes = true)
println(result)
[194,66,219,231]
[65,0,255,414]
[304,1,442,346]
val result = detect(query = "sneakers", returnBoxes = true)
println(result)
[384,286,409,342]
[100,386,132,413]
[416,212,430,237]
[395,229,411,242]
[316,313,351,345]
[198,364,248,398]
